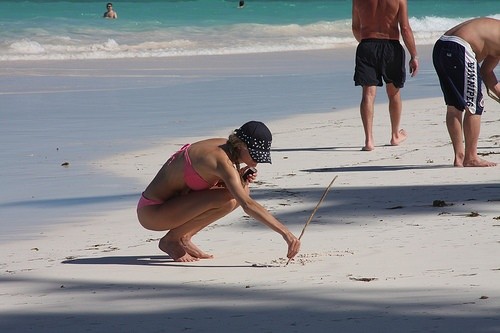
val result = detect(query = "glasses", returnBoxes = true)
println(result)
[106,6,112,8]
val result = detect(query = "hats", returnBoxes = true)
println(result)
[233,120,273,164]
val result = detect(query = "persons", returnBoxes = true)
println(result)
[352,0,419,151]
[137,121,301,262]
[237,1,245,8]
[433,18,500,167]
[104,3,117,19]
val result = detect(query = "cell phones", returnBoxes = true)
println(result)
[240,167,257,184]
